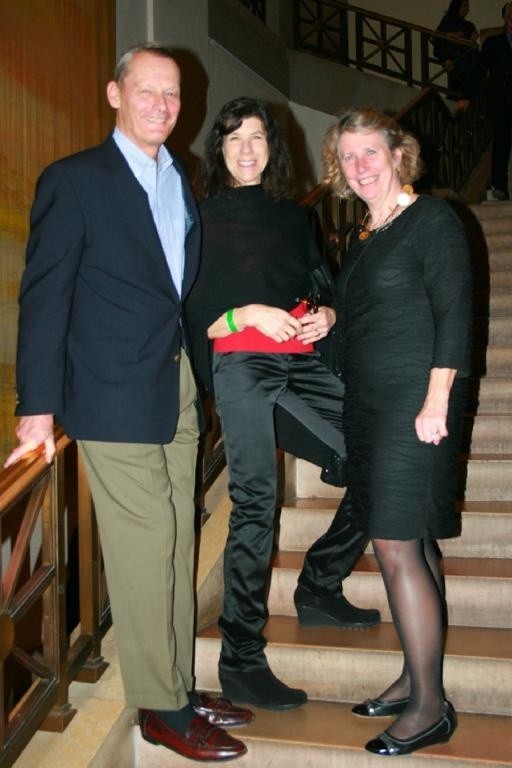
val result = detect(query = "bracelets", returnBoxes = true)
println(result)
[226,307,244,335]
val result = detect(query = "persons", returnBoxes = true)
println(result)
[316,103,493,757]
[181,94,382,711]
[2,39,256,763]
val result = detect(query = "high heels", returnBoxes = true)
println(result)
[218,667,307,710]
[294,586,381,628]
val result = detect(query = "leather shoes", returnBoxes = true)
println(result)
[351,698,410,718]
[365,700,457,755]
[139,710,247,762]
[190,692,254,728]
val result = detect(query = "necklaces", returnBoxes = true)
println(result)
[357,184,414,243]
